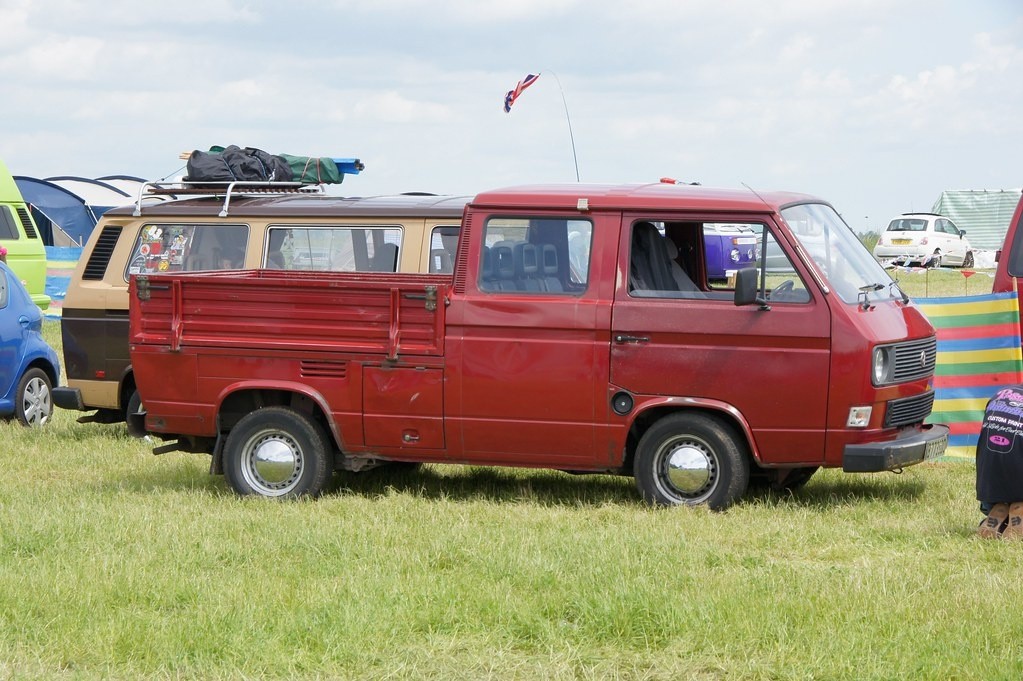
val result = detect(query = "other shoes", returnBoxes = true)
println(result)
[1003,502,1023,540]
[979,503,1009,539]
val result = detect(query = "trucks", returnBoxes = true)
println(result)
[129,184,951,509]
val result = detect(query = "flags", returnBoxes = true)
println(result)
[504,74,539,114]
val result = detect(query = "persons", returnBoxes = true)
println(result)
[374,243,399,273]
[976,386,1023,539]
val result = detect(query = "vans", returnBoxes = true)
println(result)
[41,197,601,430]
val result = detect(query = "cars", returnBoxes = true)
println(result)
[0,260,62,430]
[872,212,975,269]
[748,220,844,276]
[0,160,52,313]
[652,219,758,281]
